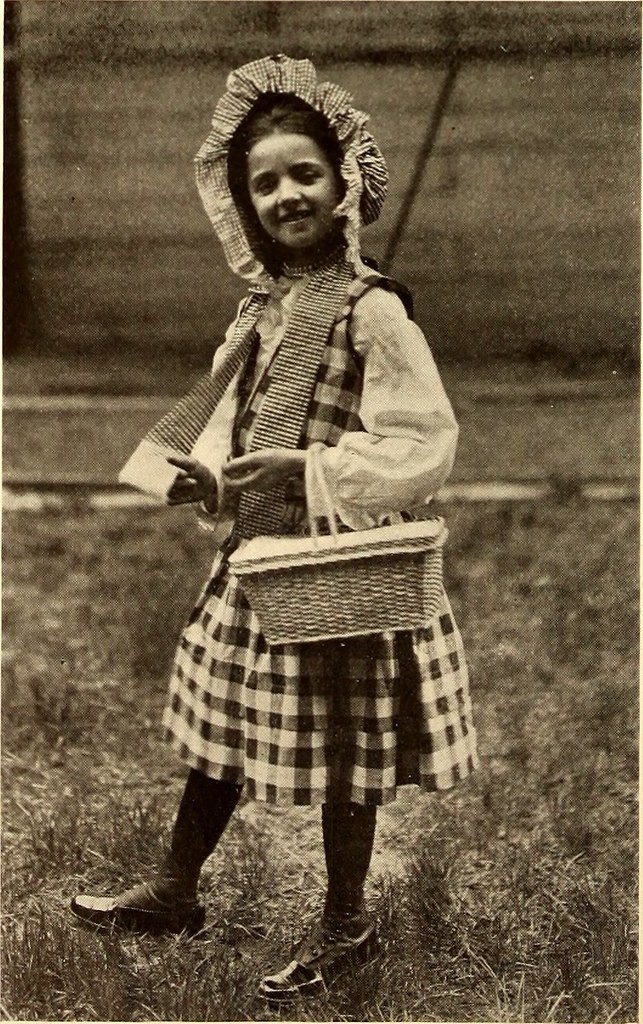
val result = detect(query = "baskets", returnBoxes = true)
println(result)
[229,442,449,644]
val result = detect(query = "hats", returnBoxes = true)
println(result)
[193,54,387,284]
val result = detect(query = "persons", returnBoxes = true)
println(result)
[71,53,478,1006]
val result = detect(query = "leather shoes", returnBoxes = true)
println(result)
[259,924,379,1002]
[70,894,206,939]
